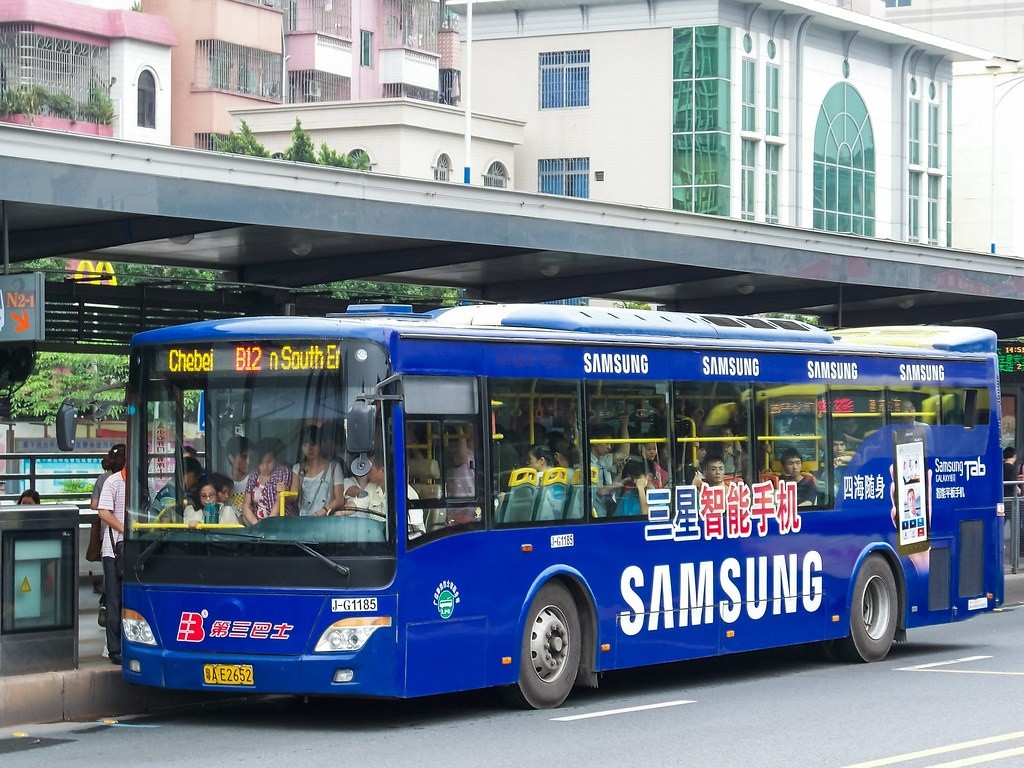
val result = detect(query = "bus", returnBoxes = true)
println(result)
[56,301,1006,712]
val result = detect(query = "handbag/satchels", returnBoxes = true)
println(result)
[86,517,101,563]
[114,542,125,580]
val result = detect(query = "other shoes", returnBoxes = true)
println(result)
[97,603,106,628]
[110,653,122,666]
[102,645,109,658]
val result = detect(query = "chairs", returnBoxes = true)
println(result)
[761,473,818,506]
[499,467,599,523]
[408,460,446,533]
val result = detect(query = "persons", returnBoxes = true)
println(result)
[1003,447,1024,557]
[91,444,127,665]
[431,405,879,524]
[893,399,934,457]
[149,422,425,540]
[17,489,40,505]
[904,489,921,519]
[903,459,920,484]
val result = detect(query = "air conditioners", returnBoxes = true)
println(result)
[303,80,322,97]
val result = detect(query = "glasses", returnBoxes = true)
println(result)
[200,494,217,498]
